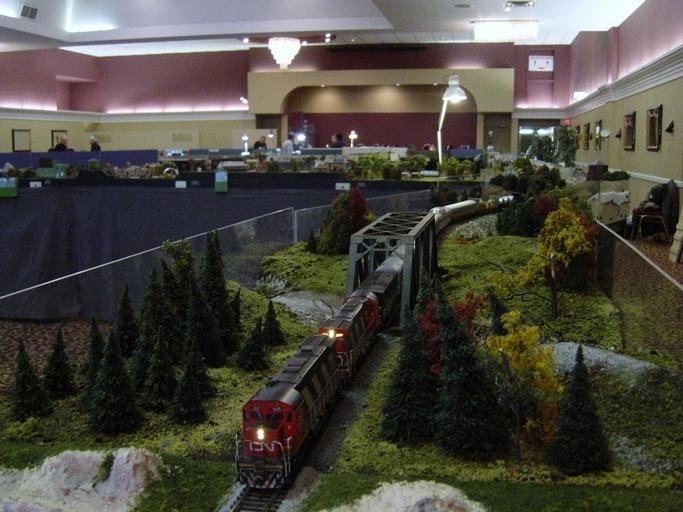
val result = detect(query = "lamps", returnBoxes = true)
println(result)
[240,132,251,156]
[268,36,301,70]
[435,74,467,166]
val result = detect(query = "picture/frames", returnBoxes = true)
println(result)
[11,128,33,154]
[583,123,590,150]
[623,111,638,151]
[595,120,602,153]
[50,129,67,148]
[646,105,663,154]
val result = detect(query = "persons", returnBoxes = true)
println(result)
[88,135,101,151]
[330,132,347,149]
[251,136,267,150]
[53,135,69,152]
[280,133,295,153]
